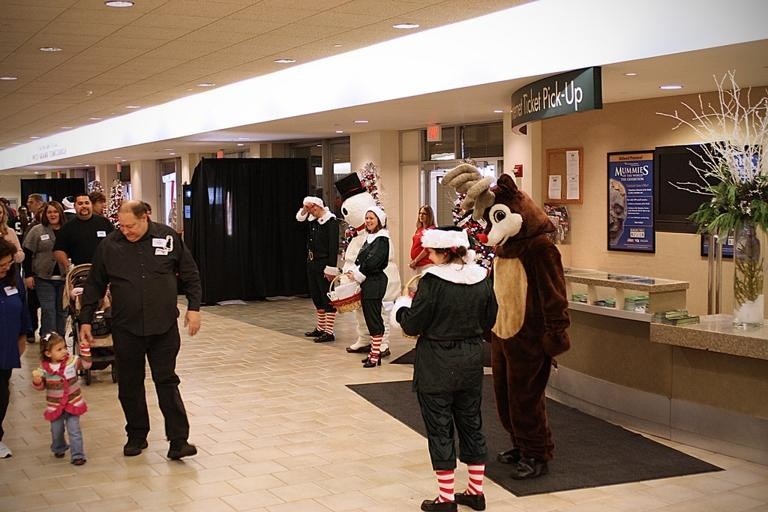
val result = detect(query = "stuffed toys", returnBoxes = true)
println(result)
[334,172,401,358]
[443,163,569,476]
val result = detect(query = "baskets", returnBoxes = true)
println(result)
[401,275,424,341]
[328,273,362,314]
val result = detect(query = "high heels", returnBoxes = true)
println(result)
[362,354,382,368]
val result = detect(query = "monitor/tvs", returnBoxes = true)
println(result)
[655,143,730,233]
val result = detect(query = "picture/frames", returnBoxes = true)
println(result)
[607,150,655,253]
[542,146,584,205]
[701,153,761,258]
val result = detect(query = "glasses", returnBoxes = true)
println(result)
[418,211,430,215]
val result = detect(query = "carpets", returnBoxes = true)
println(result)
[389,341,493,366]
[346,376,725,497]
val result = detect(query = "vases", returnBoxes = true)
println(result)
[734,218,764,331]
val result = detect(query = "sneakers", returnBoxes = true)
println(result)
[54,452,84,465]
[1,441,11,458]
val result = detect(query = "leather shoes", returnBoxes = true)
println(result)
[497,445,548,479]
[304,327,336,343]
[167,442,199,459]
[345,344,391,358]
[421,497,458,512]
[122,438,147,455]
[454,490,487,511]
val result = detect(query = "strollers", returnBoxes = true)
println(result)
[58,257,122,388]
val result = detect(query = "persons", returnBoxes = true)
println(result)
[0,188,153,362]
[0,238,32,459]
[79,200,203,460]
[407,203,436,287]
[389,225,499,511]
[609,179,627,246]
[31,330,95,465]
[348,206,390,368]
[295,187,339,342]
